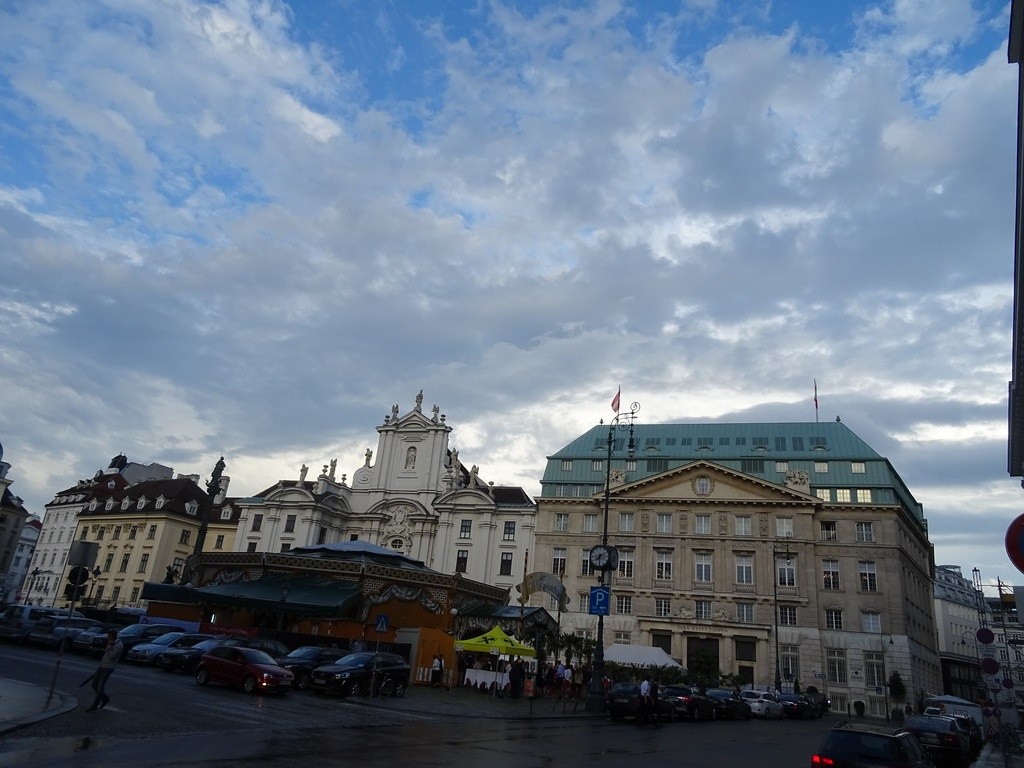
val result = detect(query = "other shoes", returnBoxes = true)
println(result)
[86,705,98,712]
[99,696,110,709]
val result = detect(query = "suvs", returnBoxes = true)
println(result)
[811,720,935,768]
[90,623,186,657]
[903,707,982,768]
[30,615,104,652]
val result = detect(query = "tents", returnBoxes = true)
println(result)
[924,695,984,740]
[603,643,688,671]
[454,625,536,696]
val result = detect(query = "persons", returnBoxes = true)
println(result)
[468,465,479,485]
[407,448,415,467]
[451,448,459,465]
[86,629,123,713]
[640,675,662,729]
[486,656,583,699]
[391,404,399,419]
[300,464,308,482]
[211,457,226,483]
[431,404,439,418]
[330,459,337,475]
[365,449,372,465]
[432,654,444,689]
[904,701,915,718]
[415,390,423,407]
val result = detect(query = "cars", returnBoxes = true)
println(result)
[195,646,294,695]
[309,651,411,697]
[73,624,125,653]
[127,632,187,668]
[161,637,345,689]
[607,683,832,722]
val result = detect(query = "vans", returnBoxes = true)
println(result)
[0,605,84,644]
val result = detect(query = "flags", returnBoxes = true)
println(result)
[611,391,620,412]
[814,385,818,410]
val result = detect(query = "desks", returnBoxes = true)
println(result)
[464,668,509,698]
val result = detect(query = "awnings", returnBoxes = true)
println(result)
[139,574,363,616]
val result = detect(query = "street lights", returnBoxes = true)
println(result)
[774,531,791,692]
[87,566,101,604]
[584,400,641,719]
[881,632,894,723]
[961,631,979,665]
[24,567,39,604]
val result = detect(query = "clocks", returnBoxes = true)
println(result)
[589,545,618,570]
